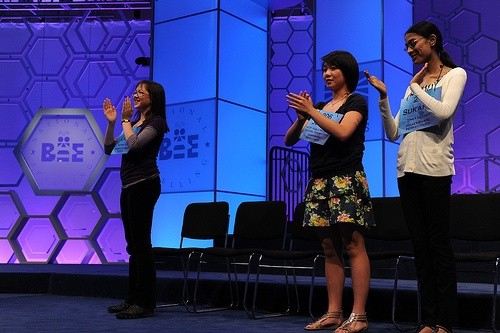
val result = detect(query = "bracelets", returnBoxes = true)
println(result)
[121,119,130,125]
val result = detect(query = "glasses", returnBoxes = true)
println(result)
[134,89,149,97]
[404,37,424,51]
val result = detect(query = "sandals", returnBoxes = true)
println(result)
[332,312,369,333]
[304,310,345,329]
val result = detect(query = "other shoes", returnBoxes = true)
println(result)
[414,324,453,333]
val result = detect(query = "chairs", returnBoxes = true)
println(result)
[155,193,500,333]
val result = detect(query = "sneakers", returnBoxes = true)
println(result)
[107,299,155,319]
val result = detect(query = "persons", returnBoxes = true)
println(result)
[363,20,470,333]
[102,79,170,320]
[282,49,372,333]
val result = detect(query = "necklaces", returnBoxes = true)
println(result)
[330,92,349,108]
